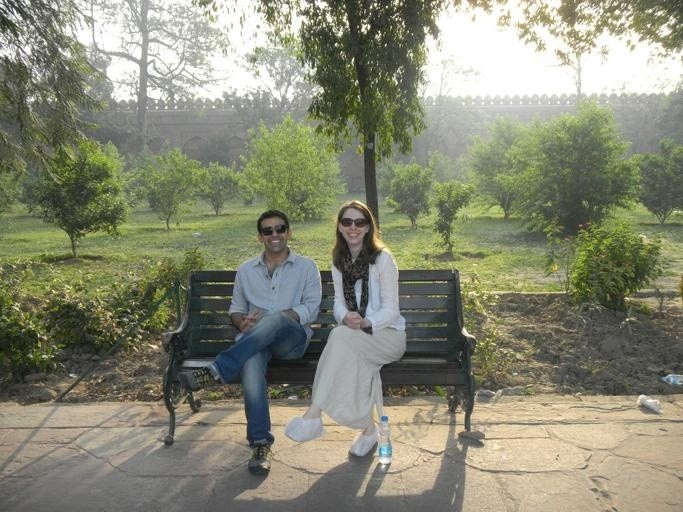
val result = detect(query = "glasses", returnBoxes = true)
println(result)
[261,224,288,236]
[339,217,367,228]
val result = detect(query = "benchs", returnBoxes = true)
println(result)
[157,270,477,446]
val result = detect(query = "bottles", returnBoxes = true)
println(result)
[377,415,392,464]
[662,373,683,385]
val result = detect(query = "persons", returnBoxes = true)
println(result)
[175,208,324,473]
[285,199,408,460]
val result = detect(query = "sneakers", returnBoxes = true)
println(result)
[284,416,323,442]
[350,426,378,458]
[179,365,217,391]
[248,445,273,473]
